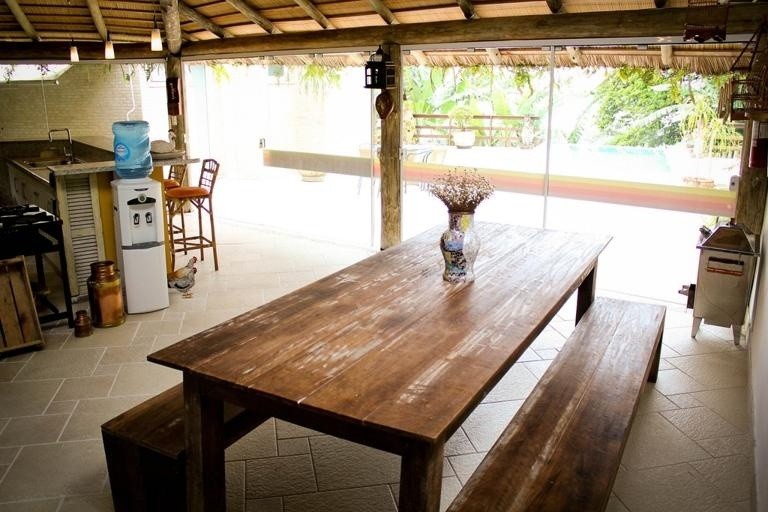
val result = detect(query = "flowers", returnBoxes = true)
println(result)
[419,161,497,211]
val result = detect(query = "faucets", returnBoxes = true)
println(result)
[49,128,74,160]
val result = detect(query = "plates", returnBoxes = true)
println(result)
[152,149,184,158]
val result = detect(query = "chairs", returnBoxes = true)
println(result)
[164,154,221,276]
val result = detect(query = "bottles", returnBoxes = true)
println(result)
[74,261,127,338]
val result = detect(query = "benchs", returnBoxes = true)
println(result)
[96,376,271,510]
[447,293,668,511]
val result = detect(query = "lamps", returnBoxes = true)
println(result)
[362,46,397,122]
[68,15,164,64]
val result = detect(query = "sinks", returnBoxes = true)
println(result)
[22,160,80,170]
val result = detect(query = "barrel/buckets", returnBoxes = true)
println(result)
[112,120,152,178]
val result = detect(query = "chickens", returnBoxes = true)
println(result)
[167,257,198,299]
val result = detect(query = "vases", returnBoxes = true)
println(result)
[438,212,480,285]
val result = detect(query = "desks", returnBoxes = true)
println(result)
[146,206,614,511]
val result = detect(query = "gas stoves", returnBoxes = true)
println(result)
[0,202,63,258]
